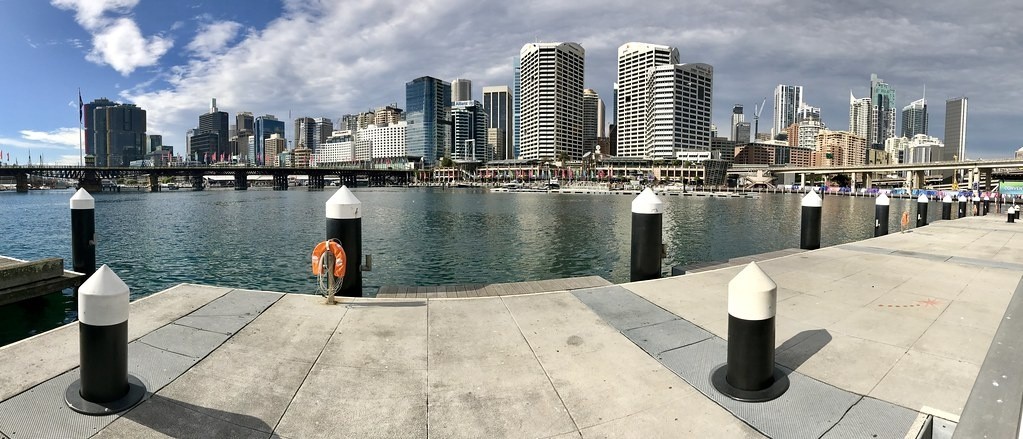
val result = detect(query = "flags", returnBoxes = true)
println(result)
[79,92,84,125]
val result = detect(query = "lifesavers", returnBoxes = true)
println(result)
[974,205,977,213]
[902,212,909,228]
[310,241,347,279]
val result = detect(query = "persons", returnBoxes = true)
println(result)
[142,160,145,167]
